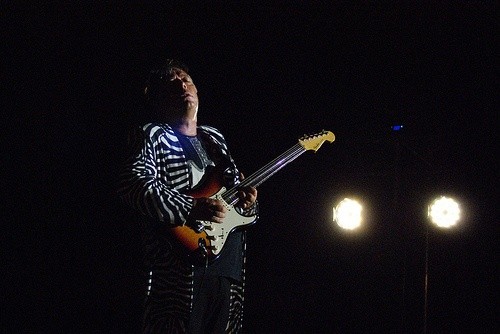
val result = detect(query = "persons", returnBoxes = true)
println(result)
[115,57,258,334]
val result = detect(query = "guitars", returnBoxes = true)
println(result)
[162,129,336,268]
